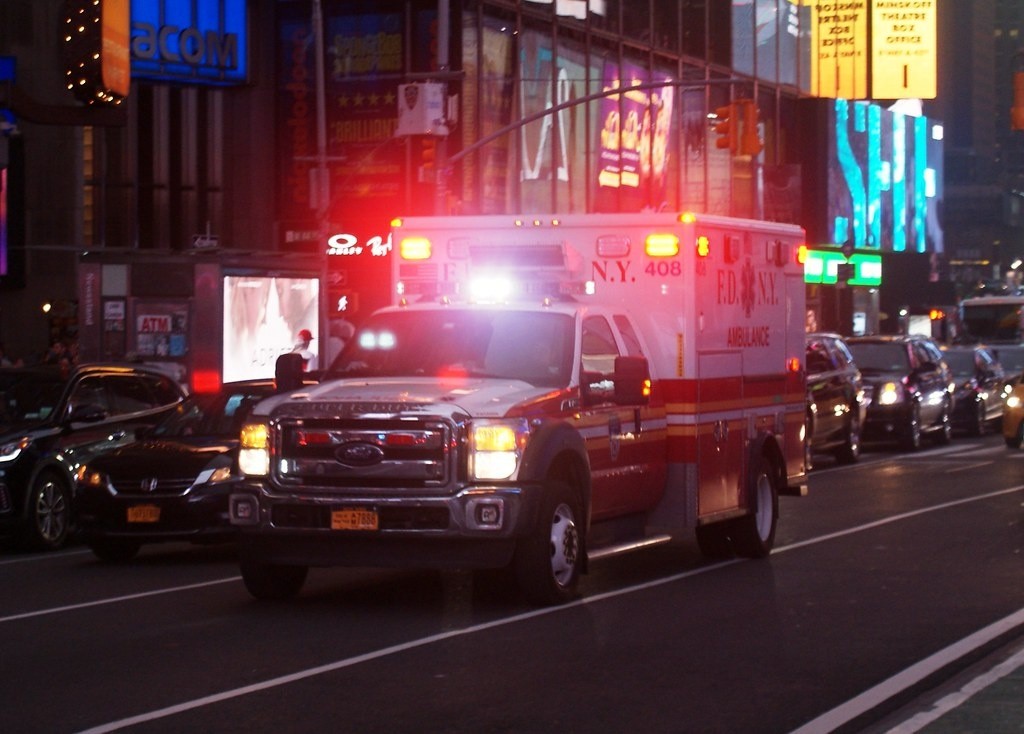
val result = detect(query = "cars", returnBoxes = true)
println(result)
[68,378,323,566]
[999,370,1024,451]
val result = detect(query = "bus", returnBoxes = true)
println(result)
[956,293,1023,345]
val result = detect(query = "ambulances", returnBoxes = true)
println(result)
[226,210,814,611]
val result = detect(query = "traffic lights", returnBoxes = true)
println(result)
[740,102,764,158]
[713,103,739,157]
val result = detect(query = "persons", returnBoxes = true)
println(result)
[225,275,316,354]
[289,327,318,373]
[0,327,81,368]
[157,336,170,356]
[602,97,666,174]
[397,85,425,132]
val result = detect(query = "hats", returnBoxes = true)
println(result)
[298,330,314,340]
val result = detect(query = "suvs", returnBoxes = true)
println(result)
[0,360,199,553]
[847,333,956,453]
[806,332,869,473]
[938,341,1010,437]
